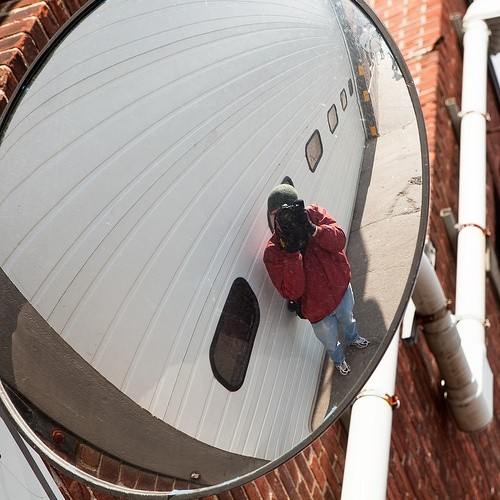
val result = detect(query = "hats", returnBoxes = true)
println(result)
[267,184,298,233]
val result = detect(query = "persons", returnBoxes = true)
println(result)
[370,33,386,61]
[263,182,371,376]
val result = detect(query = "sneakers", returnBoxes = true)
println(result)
[351,336,370,349]
[336,360,351,375]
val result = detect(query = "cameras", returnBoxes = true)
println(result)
[273,204,302,234]
